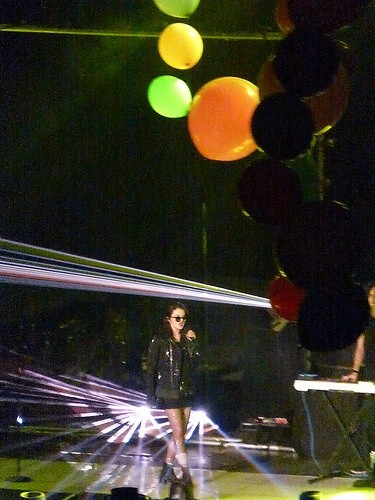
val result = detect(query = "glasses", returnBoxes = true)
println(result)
[170,315,187,322]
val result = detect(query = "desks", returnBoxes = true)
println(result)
[293,378,375,478]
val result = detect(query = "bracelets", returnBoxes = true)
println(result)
[352,370,359,373]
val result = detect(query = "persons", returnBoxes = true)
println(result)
[341,282,375,474]
[146,299,202,484]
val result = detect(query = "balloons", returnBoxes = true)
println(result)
[187,75,264,162]
[239,83,375,352]
[154,0,200,19]
[147,75,193,119]
[275,0,365,34]
[337,39,356,75]
[158,21,204,70]
[257,58,349,134]
[273,29,341,95]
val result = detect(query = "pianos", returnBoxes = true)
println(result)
[293,373,375,488]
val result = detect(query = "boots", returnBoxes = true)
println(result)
[159,463,182,484]
[181,467,193,486]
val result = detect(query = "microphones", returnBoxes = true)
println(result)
[184,325,194,343]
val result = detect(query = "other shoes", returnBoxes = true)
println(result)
[351,465,368,474]
